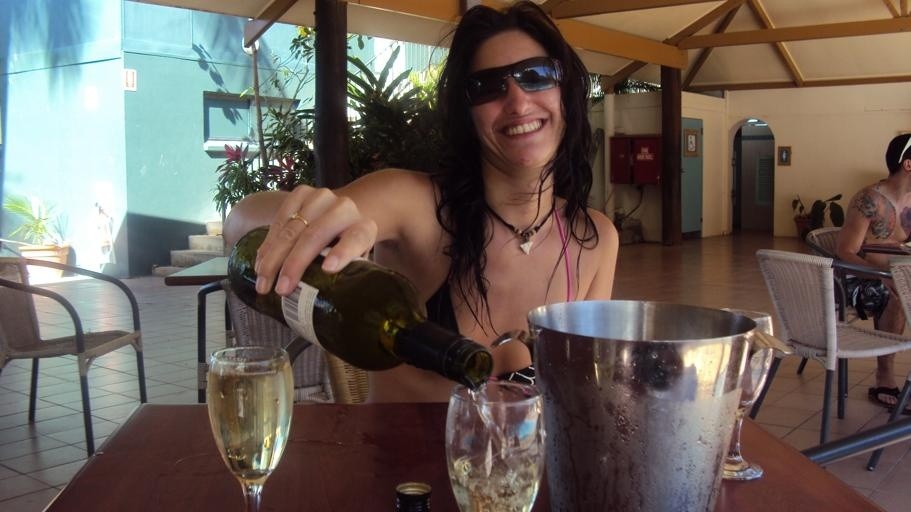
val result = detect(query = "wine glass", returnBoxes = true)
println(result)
[205,346,293,512]
[446,382,544,511]
[719,307,775,483]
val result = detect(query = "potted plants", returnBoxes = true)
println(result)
[2,195,71,283]
[792,192,844,239]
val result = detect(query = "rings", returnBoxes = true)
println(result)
[288,214,310,228]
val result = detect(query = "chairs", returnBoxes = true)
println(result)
[0,256,147,457]
[749,224,911,471]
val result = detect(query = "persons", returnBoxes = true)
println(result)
[838,133,911,405]
[222,0,619,404]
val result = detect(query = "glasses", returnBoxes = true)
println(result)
[461,57,564,107]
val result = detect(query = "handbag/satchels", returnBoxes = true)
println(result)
[834,277,891,320]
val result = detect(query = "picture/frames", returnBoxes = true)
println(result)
[684,128,699,156]
[778,145,790,165]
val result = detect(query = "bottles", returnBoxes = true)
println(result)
[227,226,493,390]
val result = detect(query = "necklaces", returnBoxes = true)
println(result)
[481,197,557,254]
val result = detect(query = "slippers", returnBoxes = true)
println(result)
[868,387,900,408]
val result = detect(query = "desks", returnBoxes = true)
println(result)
[165,257,234,403]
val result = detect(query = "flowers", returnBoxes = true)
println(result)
[212,101,316,216]
